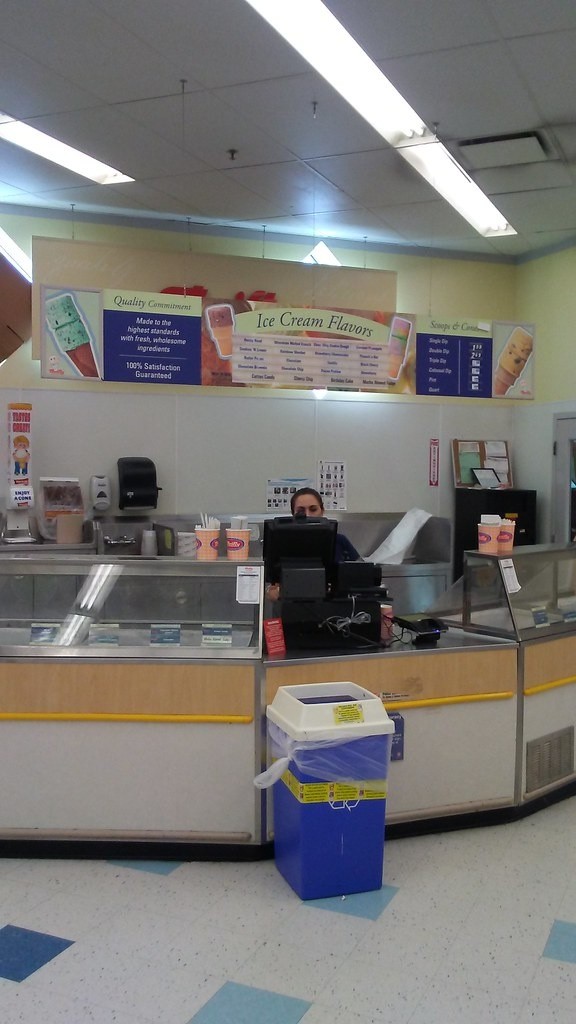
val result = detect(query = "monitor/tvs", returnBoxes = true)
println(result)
[263,514,338,586]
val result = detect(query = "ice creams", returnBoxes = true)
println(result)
[384,317,411,378]
[495,326,534,395]
[43,295,99,378]
[207,305,233,357]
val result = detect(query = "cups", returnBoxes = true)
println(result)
[497,524,515,555]
[194,528,220,560]
[142,529,158,558]
[477,523,501,555]
[380,605,393,628]
[226,528,252,560]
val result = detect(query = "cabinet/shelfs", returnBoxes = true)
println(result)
[453,488,538,584]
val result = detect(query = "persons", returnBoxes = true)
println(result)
[265,488,364,601]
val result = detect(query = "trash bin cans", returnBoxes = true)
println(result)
[264,680,396,902]
